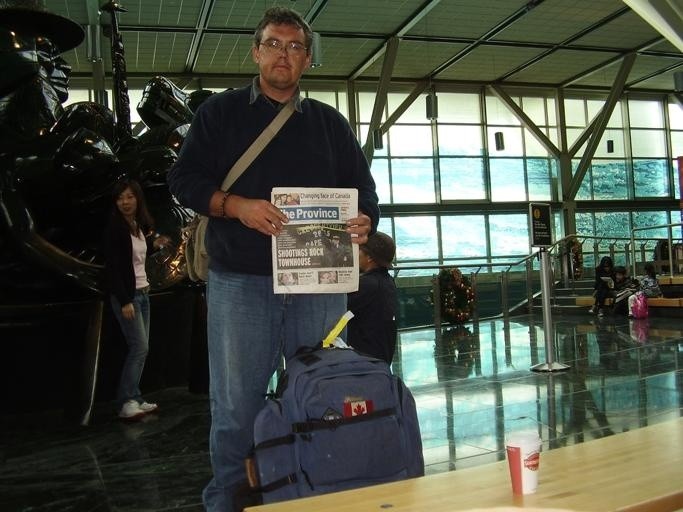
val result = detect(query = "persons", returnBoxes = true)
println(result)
[1,0,85,103]
[349,233,398,367]
[589,256,613,317]
[321,233,347,267]
[639,263,661,297]
[168,7,380,512]
[613,266,630,289]
[110,178,172,419]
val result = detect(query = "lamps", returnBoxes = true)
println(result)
[373,128,384,150]
[424,1,439,121]
[607,139,614,153]
[495,131,505,151]
[308,0,322,69]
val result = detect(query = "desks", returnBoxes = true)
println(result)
[243,416,683,512]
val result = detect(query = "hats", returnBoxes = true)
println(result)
[360,231,396,269]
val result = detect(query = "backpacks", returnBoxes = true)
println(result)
[246,342,425,505]
[628,291,649,318]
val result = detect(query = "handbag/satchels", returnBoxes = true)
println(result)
[182,94,304,283]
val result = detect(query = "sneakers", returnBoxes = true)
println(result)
[140,401,159,413]
[117,398,147,419]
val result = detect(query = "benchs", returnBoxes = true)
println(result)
[576,294,683,314]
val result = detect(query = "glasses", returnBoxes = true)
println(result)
[258,39,308,55]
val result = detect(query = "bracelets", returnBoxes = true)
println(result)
[220,191,234,223]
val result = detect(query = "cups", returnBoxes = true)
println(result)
[506,439,542,495]
